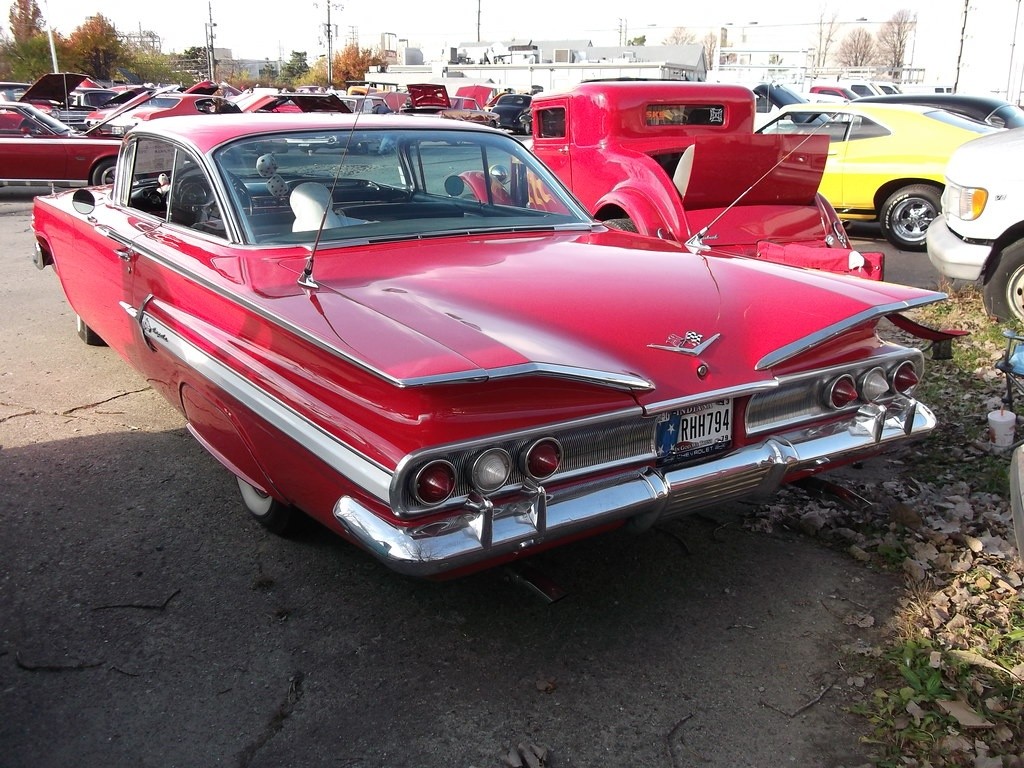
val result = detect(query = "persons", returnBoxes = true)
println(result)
[277,87,287,94]
[246,88,254,93]
[318,85,337,96]
[212,90,231,107]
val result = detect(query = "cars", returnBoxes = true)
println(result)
[455,79,972,344]
[0,87,156,188]
[0,60,1024,254]
[925,125,1024,330]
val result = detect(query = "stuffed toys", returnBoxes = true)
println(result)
[156,173,182,216]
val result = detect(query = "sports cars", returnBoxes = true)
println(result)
[30,110,949,584]
